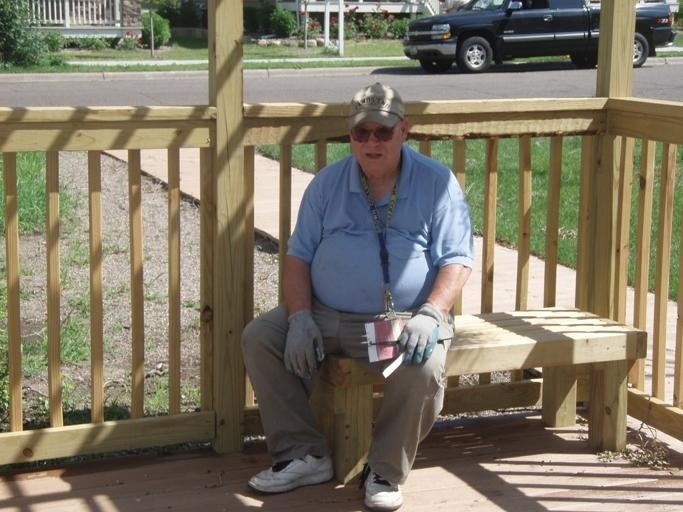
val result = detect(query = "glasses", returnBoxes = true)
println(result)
[351,127,395,143]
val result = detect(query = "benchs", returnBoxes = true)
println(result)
[309,306,646,484]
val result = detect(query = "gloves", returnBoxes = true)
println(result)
[396,303,443,366]
[282,309,325,380]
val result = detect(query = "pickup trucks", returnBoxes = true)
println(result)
[401,0,676,70]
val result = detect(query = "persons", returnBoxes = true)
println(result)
[240,83,474,512]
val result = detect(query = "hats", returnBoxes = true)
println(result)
[348,81,404,128]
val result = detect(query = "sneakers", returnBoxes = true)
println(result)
[363,464,403,511]
[247,453,334,495]
